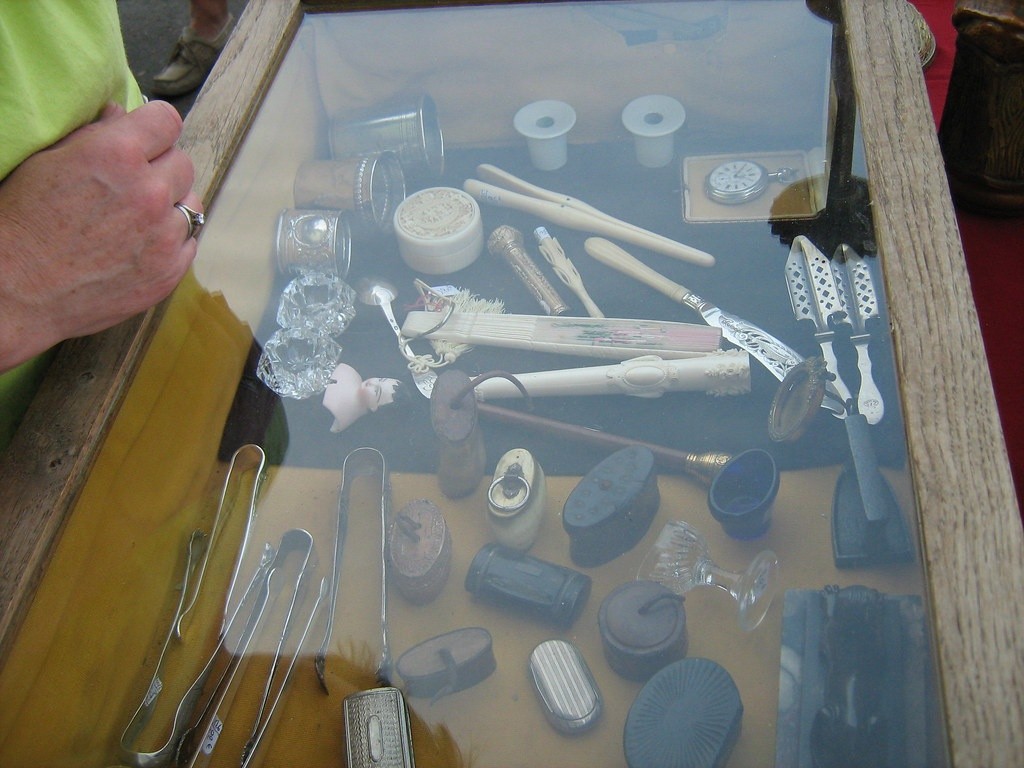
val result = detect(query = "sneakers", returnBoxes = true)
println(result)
[150,12,235,97]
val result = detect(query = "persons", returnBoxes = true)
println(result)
[0,0,207,378]
[152,0,232,95]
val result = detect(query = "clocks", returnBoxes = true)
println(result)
[705,160,799,204]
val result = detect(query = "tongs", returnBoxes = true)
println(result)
[313,446,395,695]
[185,566,332,768]
[116,527,283,768]
[171,526,318,768]
[174,444,269,649]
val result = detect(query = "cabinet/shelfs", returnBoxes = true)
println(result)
[0,0,1024,768]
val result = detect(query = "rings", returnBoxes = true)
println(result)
[175,203,206,239]
